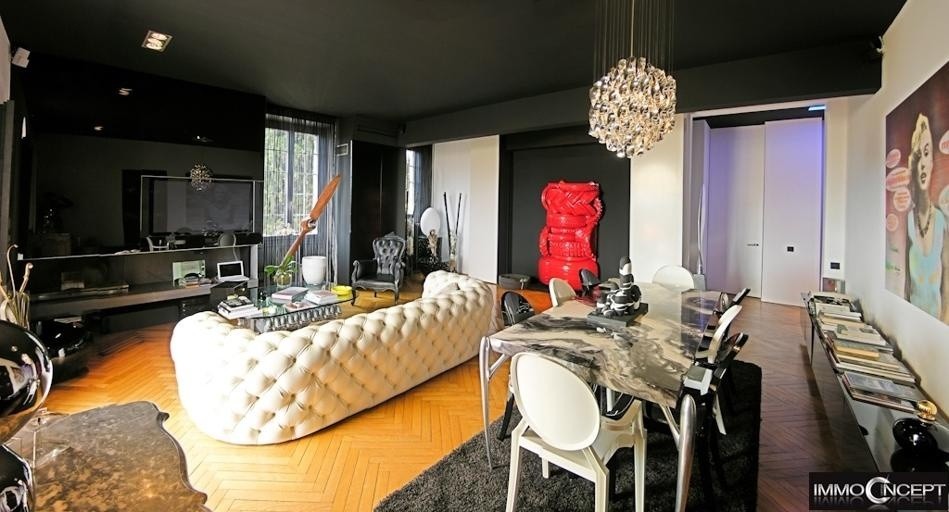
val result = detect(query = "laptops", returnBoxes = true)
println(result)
[216,260,249,283]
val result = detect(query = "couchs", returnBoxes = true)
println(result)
[169,270,494,445]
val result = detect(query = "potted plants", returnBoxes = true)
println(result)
[264,255,296,287]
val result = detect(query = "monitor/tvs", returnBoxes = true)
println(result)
[140,175,264,248]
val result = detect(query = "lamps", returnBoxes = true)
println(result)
[589,0,677,160]
[189,161,214,191]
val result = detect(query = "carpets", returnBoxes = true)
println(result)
[372,360,760,512]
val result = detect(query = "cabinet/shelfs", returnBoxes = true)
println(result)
[15,245,259,331]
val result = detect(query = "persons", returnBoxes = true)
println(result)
[902,113,949,326]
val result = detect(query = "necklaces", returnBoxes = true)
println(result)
[912,205,932,239]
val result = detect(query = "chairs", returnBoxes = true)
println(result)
[350,233,406,305]
[653,265,694,289]
[579,269,600,297]
[713,285,750,317]
[505,351,647,512]
[696,305,743,364]
[676,332,748,512]
[549,278,577,308]
[499,292,535,440]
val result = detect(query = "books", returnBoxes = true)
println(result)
[217,295,264,320]
[801,290,917,413]
[270,287,338,306]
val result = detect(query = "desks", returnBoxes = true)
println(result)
[0,402,211,512]
[803,292,947,511]
[479,277,728,468]
